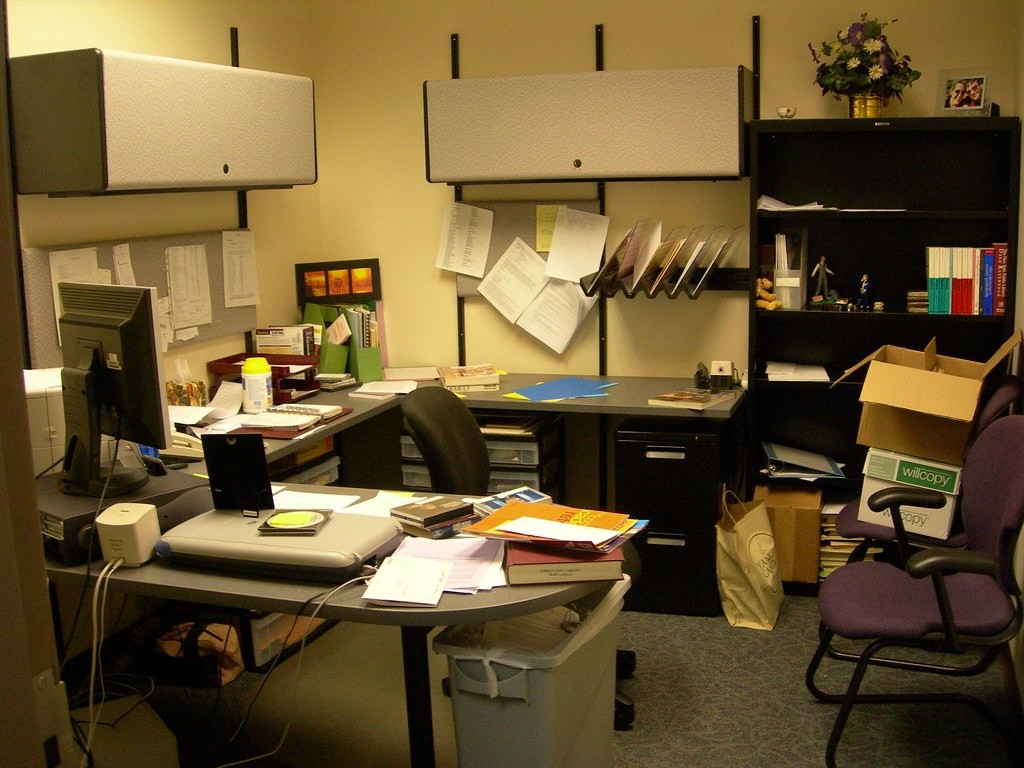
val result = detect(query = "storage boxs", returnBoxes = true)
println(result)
[823,331,1020,542]
[749,483,821,587]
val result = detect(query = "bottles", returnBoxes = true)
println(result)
[241,357,273,413]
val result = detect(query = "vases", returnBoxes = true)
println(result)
[848,92,888,120]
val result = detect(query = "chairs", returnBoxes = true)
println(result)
[819,375,1023,678]
[807,416,1024,767]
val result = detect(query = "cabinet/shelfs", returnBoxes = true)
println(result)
[748,118,1020,596]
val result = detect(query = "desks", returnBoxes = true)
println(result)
[29,368,750,768]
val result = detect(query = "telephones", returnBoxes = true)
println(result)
[158,432,205,461]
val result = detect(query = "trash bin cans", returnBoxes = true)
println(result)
[431,573,631,768]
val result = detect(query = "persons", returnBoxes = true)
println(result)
[859,275,869,308]
[811,255,834,301]
[944,79,984,109]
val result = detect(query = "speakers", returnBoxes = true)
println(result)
[95,503,161,566]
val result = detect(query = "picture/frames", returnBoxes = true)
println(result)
[932,59,995,117]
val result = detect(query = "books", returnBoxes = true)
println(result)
[389,486,649,586]
[926,242,1010,317]
[240,306,541,435]
[819,499,884,582]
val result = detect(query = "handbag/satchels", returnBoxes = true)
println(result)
[714,489,786,631]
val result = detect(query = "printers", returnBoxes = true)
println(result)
[24,368,147,475]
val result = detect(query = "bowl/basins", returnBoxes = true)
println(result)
[777,107,797,119]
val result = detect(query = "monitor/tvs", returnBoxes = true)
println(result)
[56,282,172,499]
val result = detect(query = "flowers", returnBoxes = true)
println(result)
[809,12,921,105]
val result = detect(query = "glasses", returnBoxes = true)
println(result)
[966,83,979,94]
[954,89,965,94]
